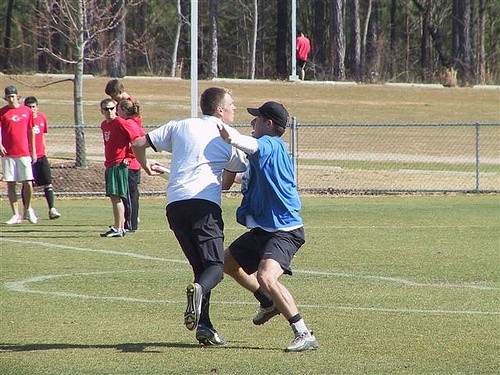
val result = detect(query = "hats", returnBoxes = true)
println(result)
[247,101,289,129]
[5,85,18,94]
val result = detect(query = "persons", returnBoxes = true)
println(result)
[216,101,319,353]
[0,85,38,224]
[101,79,147,235]
[21,96,61,219]
[131,87,247,345]
[297,30,311,81]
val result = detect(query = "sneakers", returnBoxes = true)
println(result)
[183,283,202,331]
[284,330,319,352]
[253,304,281,326]
[196,325,228,347]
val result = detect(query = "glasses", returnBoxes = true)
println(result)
[102,107,115,111]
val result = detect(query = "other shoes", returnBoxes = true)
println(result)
[100,225,126,237]
[48,208,61,219]
[4,215,21,225]
[26,207,37,224]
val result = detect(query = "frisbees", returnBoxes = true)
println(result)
[152,164,170,180]
